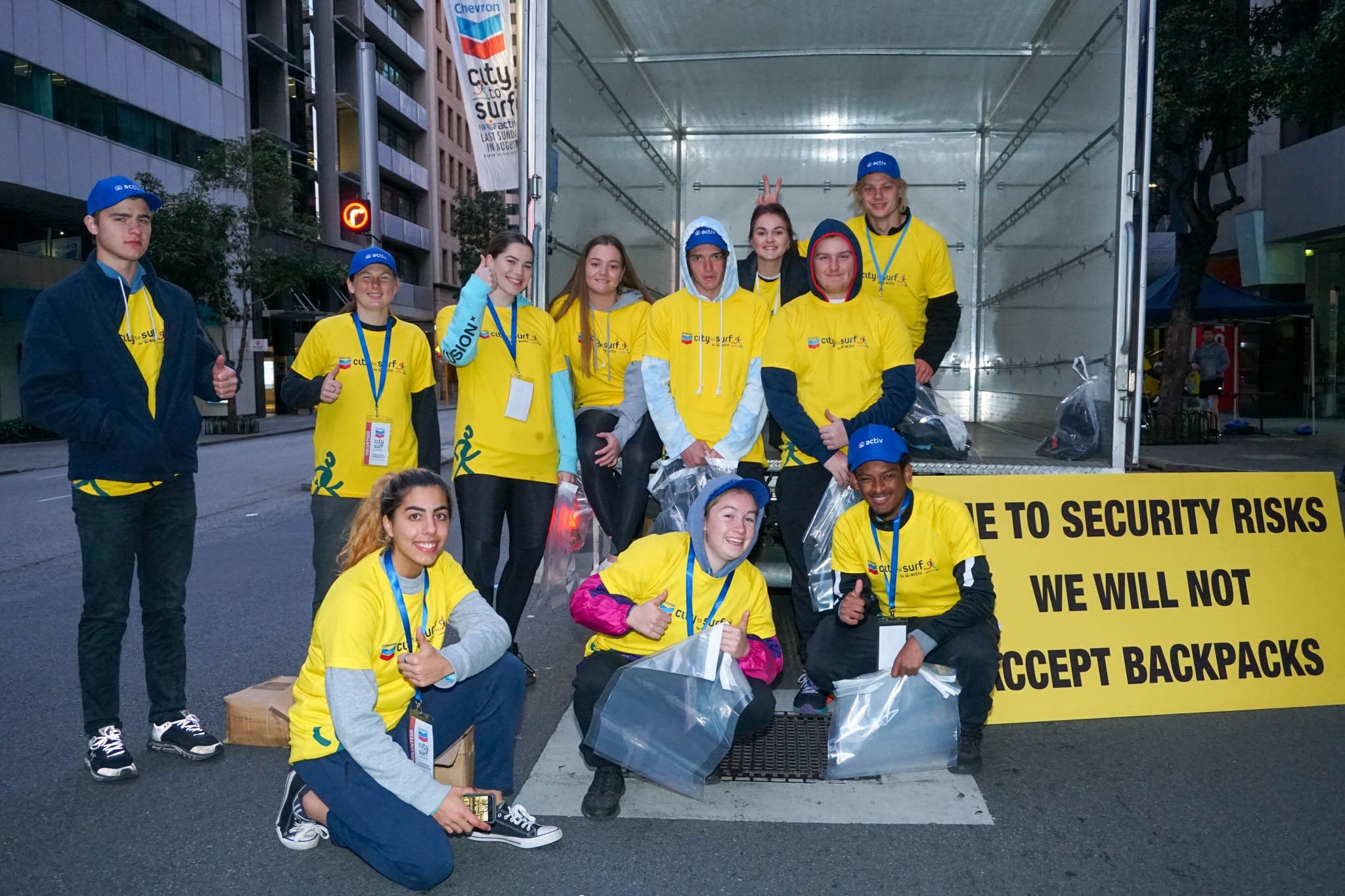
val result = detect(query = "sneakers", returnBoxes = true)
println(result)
[466,799,563,848]
[947,729,984,775]
[276,769,331,851]
[83,724,140,783]
[792,669,834,716]
[146,714,226,761]
[581,765,627,822]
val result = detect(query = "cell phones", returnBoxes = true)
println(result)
[461,793,496,825]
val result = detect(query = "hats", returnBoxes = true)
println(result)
[87,174,164,216]
[857,151,901,180]
[707,477,770,509]
[349,246,401,278]
[846,422,909,470]
[685,227,730,257]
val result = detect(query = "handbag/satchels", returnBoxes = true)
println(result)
[650,457,738,537]
[1223,419,1249,435]
[894,382,974,463]
[1034,354,1102,461]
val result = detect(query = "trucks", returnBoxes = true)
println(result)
[522,1,1156,574]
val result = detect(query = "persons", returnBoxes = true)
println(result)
[757,153,960,396]
[434,230,578,685]
[548,234,663,555]
[15,175,244,782]
[274,468,566,894]
[737,203,812,460]
[571,473,784,821]
[760,218,917,717]
[805,425,1000,776]
[642,215,771,564]
[1191,323,1230,438]
[282,246,442,620]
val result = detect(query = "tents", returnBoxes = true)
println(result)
[1146,264,1315,436]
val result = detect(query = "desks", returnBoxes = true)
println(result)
[1143,390,1277,438]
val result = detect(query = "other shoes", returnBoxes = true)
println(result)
[507,643,537,686]
[1204,428,1221,439]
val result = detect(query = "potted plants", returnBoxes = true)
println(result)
[1313,343,1338,419]
